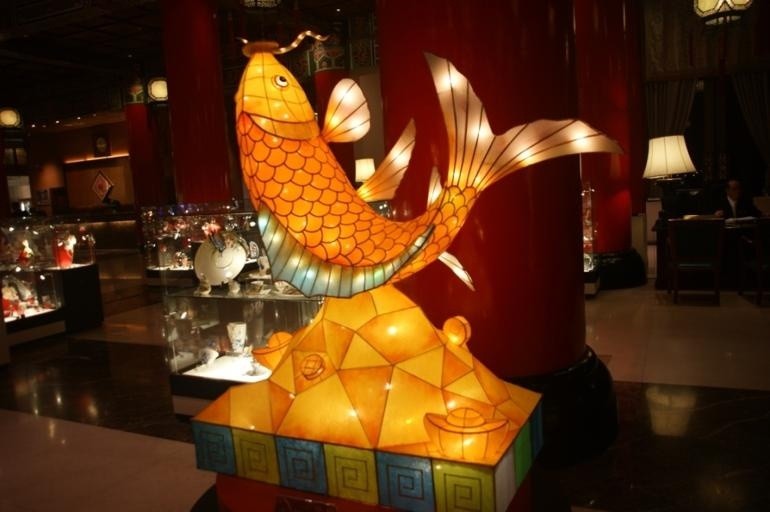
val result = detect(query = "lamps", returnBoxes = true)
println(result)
[642,137,698,231]
[696,0,756,25]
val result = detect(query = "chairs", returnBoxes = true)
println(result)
[653,211,769,305]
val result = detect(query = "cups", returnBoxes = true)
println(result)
[226,321,247,355]
[257,254,271,277]
[250,280,264,292]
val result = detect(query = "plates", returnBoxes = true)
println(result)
[246,288,272,296]
[274,290,305,299]
[249,273,272,280]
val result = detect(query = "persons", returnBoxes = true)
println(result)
[705,177,770,219]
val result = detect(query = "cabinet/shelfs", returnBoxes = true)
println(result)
[0,223,103,363]
[157,213,326,422]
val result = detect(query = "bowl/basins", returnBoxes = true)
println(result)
[194,238,247,284]
